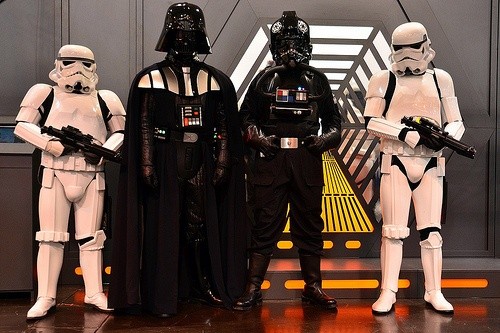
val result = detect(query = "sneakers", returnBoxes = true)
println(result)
[84,292,114,312]
[372,288,396,314]
[424,290,454,313]
[27,296,56,319]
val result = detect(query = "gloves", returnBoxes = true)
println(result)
[84,151,102,165]
[141,166,158,187]
[416,131,425,147]
[245,124,281,160]
[425,137,445,152]
[213,167,229,185]
[307,127,341,155]
[60,141,80,156]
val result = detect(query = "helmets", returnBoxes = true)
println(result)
[155,2,213,54]
[391,22,435,76]
[49,45,98,93]
[269,11,313,66]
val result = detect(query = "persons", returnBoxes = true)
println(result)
[107,2,240,320]
[229,10,342,310]
[363,21,466,315]
[13,45,128,321]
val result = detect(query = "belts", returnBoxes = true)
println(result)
[273,137,307,150]
[166,130,209,144]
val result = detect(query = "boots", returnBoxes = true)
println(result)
[233,252,271,310]
[298,255,338,308]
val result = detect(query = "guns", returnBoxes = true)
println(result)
[40,125,124,166]
[400,116,477,160]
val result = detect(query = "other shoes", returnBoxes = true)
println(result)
[156,312,171,318]
[191,285,225,306]
